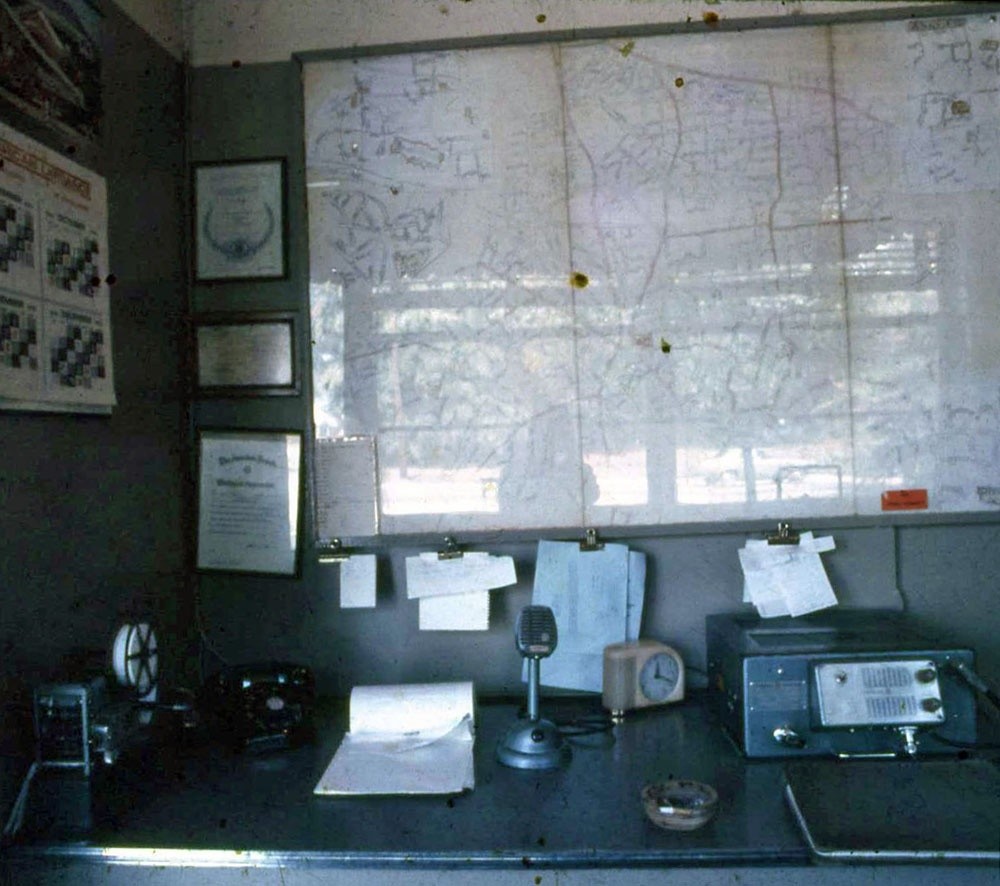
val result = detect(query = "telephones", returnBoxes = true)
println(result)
[207,663,310,751]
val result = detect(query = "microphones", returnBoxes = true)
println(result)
[516,606,558,721]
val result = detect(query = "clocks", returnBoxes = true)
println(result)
[631,638,685,709]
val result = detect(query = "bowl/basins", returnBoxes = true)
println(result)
[641,779,718,832]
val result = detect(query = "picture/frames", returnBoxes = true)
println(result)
[190,155,290,284]
[189,309,303,402]
[187,424,305,579]
